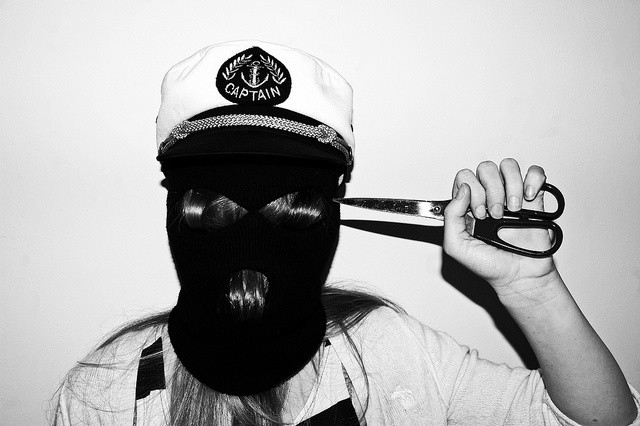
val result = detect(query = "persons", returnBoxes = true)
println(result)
[45,39,640,425]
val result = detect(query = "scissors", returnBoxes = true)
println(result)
[332,182,565,258]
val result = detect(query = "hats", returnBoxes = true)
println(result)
[154,36,357,185]
